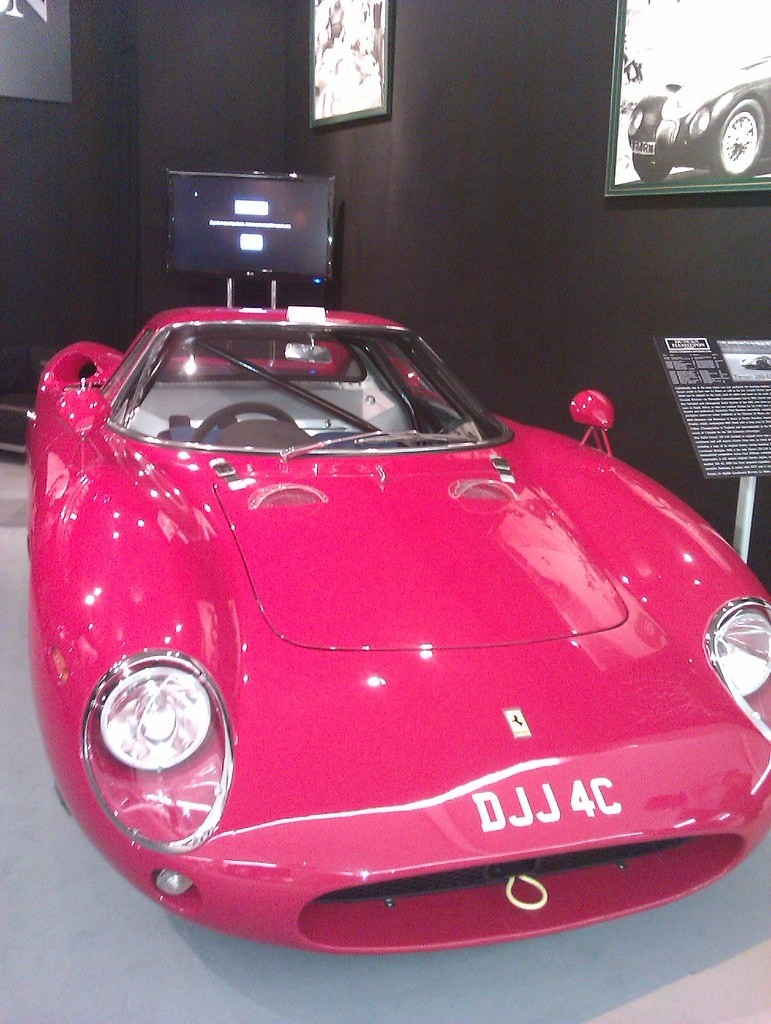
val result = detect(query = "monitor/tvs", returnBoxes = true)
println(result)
[308,0,396,131]
[164,169,336,282]
[603,0,770,197]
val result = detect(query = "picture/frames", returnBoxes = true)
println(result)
[309,0,393,131]
[603,2,770,200]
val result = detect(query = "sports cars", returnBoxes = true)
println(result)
[30,299,771,951]
[625,58,771,186]
[739,353,770,372]
